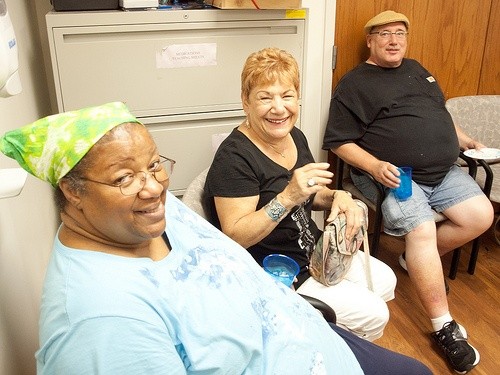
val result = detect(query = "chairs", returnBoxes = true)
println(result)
[181,91,500,325]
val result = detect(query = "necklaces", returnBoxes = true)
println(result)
[267,143,286,158]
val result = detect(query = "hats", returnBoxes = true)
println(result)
[363,10,409,34]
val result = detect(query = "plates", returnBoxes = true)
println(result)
[463,147,500,159]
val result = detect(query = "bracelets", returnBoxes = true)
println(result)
[265,196,290,223]
[332,190,352,201]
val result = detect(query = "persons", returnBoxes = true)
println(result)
[322,10,494,375]
[203,48,397,342]
[0,101,432,375]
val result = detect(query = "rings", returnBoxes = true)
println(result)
[307,178,315,186]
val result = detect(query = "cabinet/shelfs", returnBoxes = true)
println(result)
[44,7,309,201]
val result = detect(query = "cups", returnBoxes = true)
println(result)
[391,166,412,201]
[262,254,300,288]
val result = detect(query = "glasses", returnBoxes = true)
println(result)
[368,30,408,38]
[75,155,176,195]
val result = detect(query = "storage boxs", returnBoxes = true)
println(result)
[204,0,302,10]
[50,0,120,11]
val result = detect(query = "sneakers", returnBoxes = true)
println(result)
[400,249,450,294]
[429,318,480,375]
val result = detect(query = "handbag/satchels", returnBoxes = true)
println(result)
[310,199,368,287]
